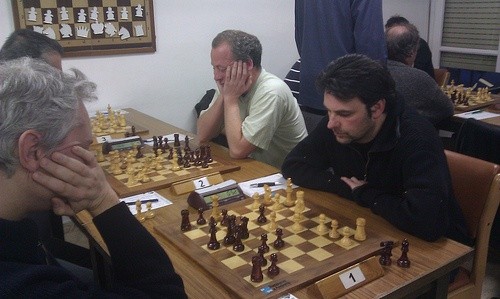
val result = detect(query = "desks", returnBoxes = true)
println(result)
[69,108,476,299]
[453,103,500,133]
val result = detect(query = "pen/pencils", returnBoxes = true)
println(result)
[472,110,484,114]
[94,112,109,114]
[125,199,158,205]
[251,182,284,187]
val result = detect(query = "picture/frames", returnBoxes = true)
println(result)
[10,0,156,58]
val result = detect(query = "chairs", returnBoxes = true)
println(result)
[452,119,499,166]
[444,149,500,299]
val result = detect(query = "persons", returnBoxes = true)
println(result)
[0,28,64,72]
[0,55,189,299]
[196,30,309,170]
[283,0,455,124]
[282,54,470,289]
